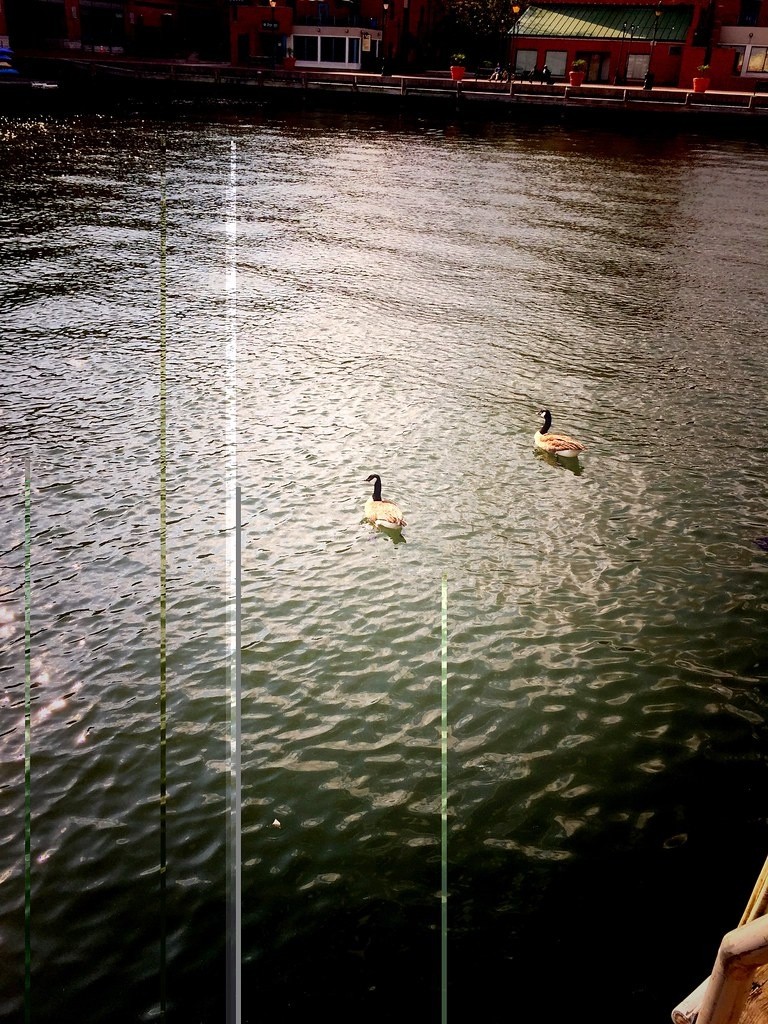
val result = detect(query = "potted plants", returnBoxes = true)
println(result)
[569,60,587,87]
[283,48,296,69]
[693,64,710,92]
[450,54,466,81]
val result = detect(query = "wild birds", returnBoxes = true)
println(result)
[534,410,588,457]
[361,473,407,529]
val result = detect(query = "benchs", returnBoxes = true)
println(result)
[476,67,553,84]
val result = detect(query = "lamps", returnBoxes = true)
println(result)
[748,33,754,38]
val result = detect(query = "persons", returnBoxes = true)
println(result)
[489,62,552,84]
